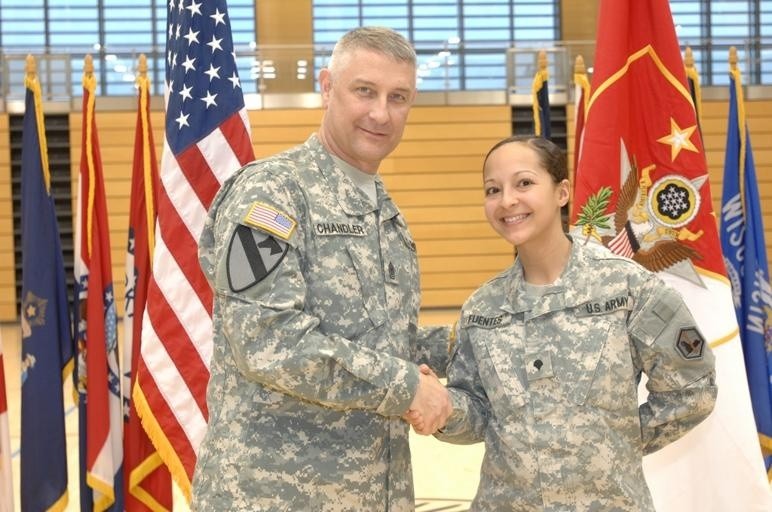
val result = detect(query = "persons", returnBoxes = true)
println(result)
[194,29,463,512]
[405,134,718,511]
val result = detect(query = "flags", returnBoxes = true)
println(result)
[531,72,552,139]
[71,73,125,511]
[19,72,75,510]
[573,73,590,202]
[125,1,257,512]
[121,74,158,421]
[570,0,731,284]
[718,71,772,482]
